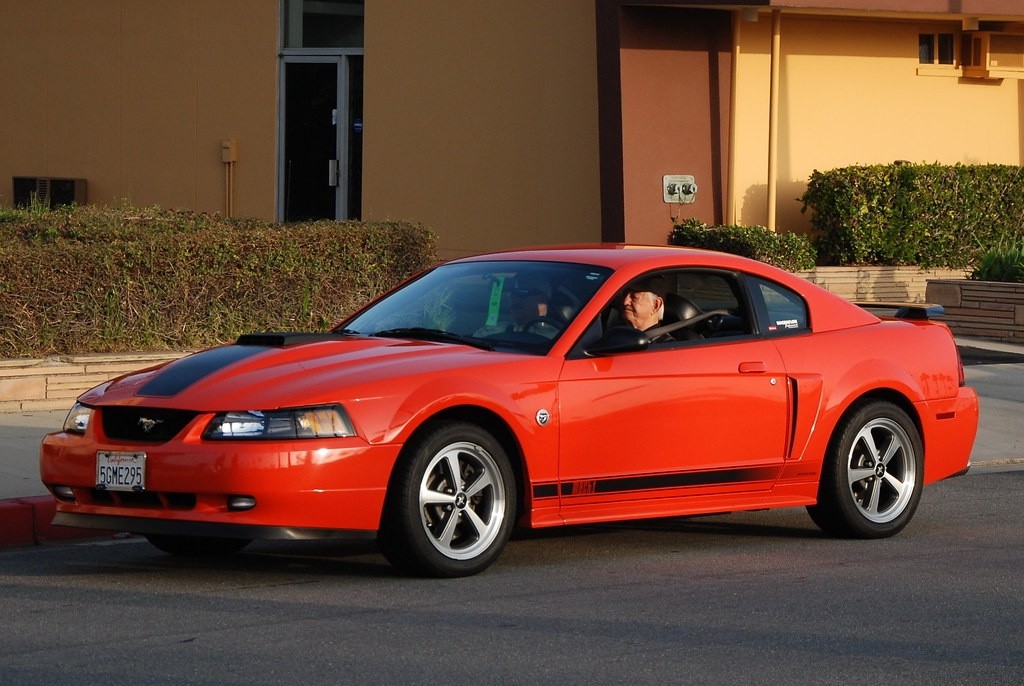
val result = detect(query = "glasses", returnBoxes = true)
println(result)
[510,286,540,298]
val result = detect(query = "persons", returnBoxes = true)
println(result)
[471,273,559,338]
[591,275,678,344]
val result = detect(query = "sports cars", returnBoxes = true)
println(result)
[35,234,981,579]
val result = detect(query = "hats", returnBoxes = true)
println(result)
[629,272,667,301]
